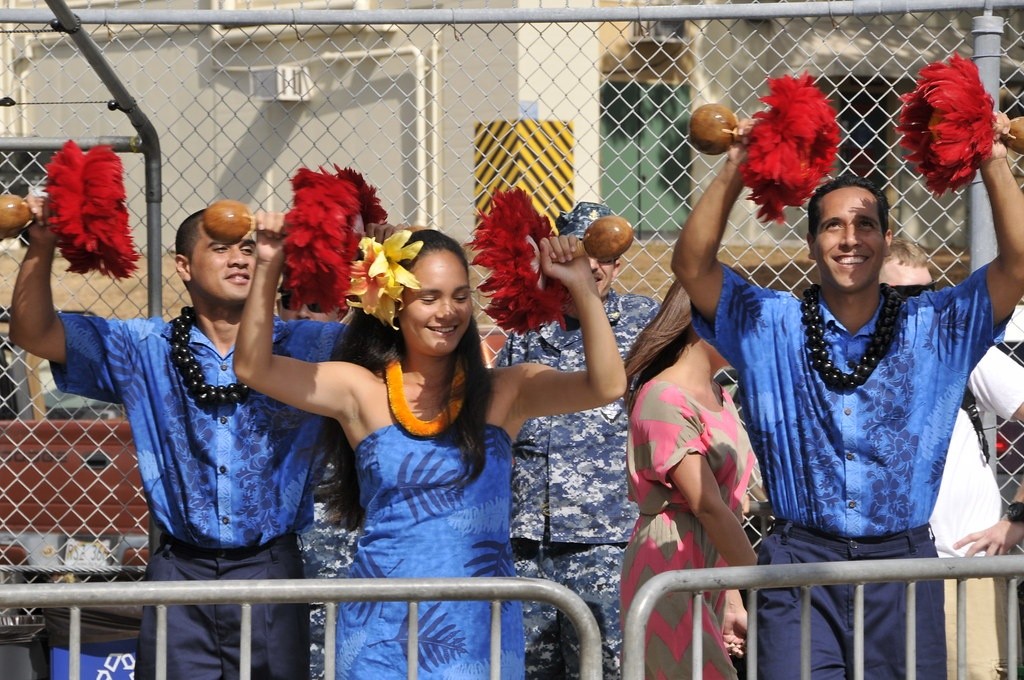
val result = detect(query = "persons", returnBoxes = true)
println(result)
[232,214,626,680]
[620,264,767,680]
[671,110,1024,679]
[879,256,1024,680]
[9,196,349,680]
[493,202,661,680]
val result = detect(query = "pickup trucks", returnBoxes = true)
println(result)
[0,418,153,577]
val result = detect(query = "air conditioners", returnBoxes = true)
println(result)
[249,66,311,102]
[630,19,686,44]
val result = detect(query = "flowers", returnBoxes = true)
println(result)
[894,52,1023,197]
[204,164,389,313]
[466,187,633,336]
[342,229,425,331]
[1,139,141,282]
[690,69,842,224]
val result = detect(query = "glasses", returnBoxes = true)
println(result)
[279,294,323,313]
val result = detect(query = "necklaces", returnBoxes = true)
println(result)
[801,280,902,388]
[171,306,251,405]
[385,360,465,437]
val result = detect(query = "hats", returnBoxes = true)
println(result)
[554,202,619,240]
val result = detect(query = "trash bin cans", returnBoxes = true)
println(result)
[0,612,46,680]
[48,608,147,680]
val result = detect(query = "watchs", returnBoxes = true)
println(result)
[1007,501,1024,521]
